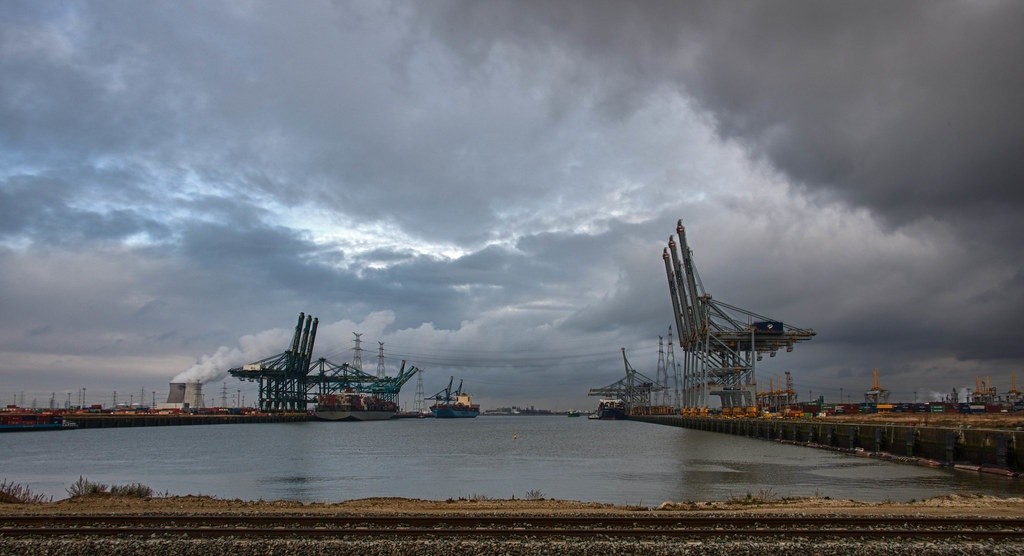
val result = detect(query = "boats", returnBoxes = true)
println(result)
[598,399,626,419]
[0,415,79,431]
[430,403,480,418]
[314,393,398,421]
[568,412,580,417]
[589,414,599,419]
